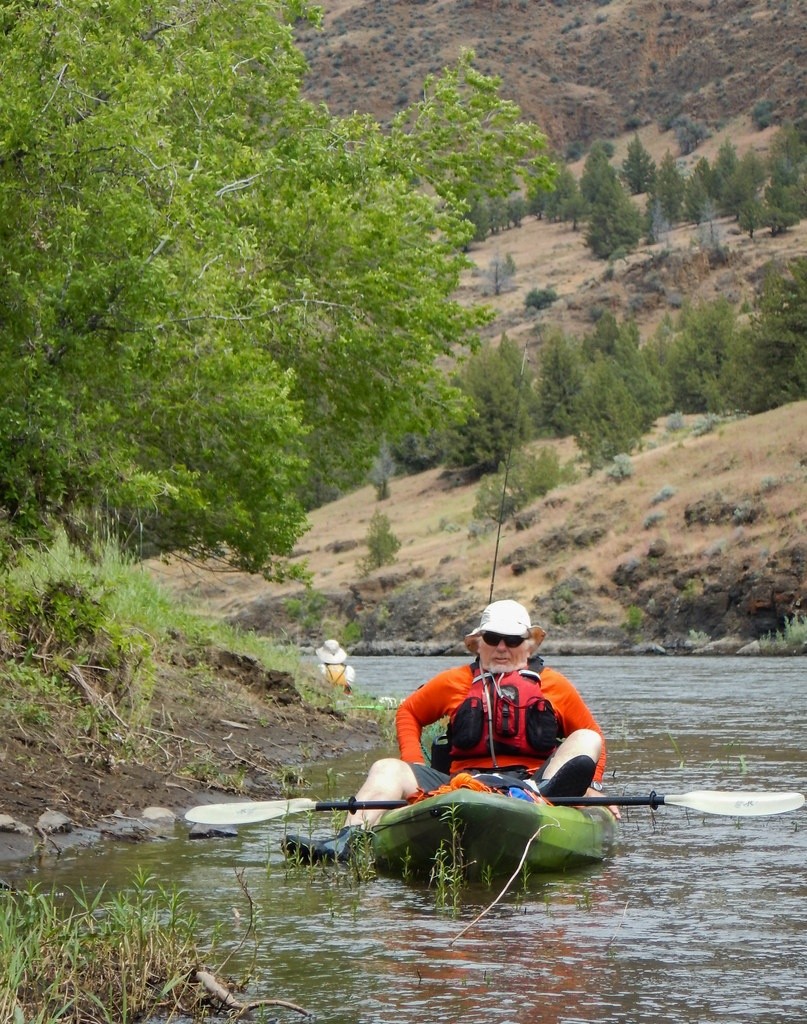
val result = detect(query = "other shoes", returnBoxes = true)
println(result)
[538,754,595,806]
[281,825,367,864]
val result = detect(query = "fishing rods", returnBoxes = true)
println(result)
[487,337,532,607]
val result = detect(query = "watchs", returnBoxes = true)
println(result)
[589,781,603,791]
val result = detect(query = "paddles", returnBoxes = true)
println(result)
[183,786,806,827]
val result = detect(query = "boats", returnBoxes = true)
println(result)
[354,788,618,881]
[330,696,405,720]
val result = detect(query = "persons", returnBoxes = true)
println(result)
[315,639,355,694]
[281,599,621,865]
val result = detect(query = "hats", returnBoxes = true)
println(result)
[465,600,547,655]
[315,639,347,664]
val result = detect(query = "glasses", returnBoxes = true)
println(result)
[481,632,529,648]
